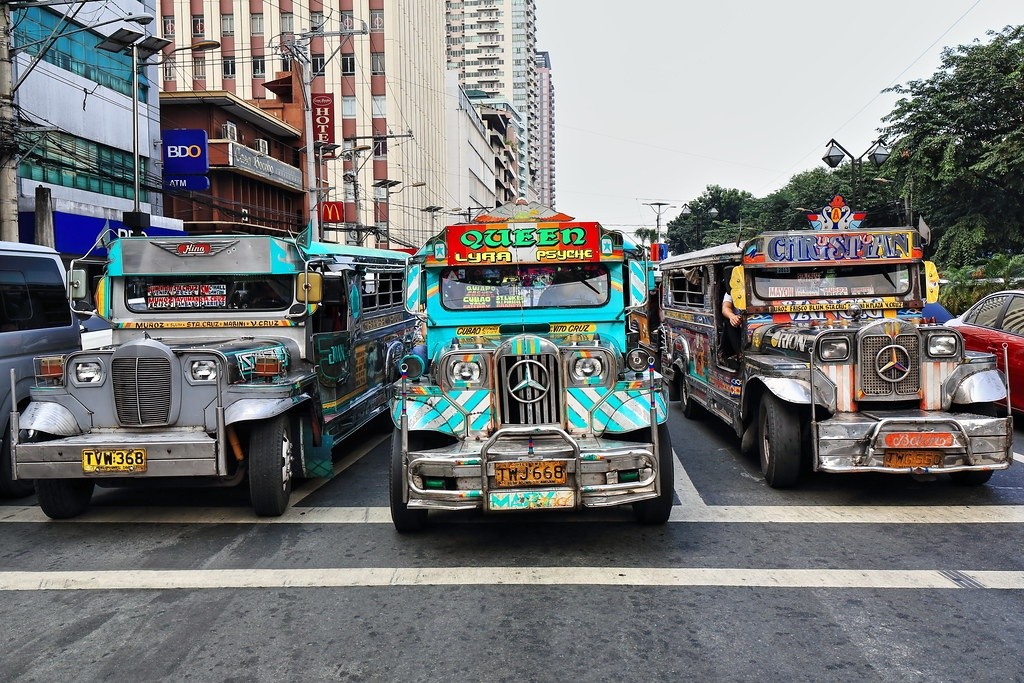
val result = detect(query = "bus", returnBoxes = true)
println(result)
[9,230,415,520]
[389,197,677,524]
[658,227,1013,491]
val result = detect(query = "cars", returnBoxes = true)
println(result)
[944,289,1024,414]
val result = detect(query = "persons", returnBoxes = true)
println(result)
[722,292,742,350]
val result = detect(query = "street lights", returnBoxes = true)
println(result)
[681,203,719,251]
[822,136,892,210]
[373,178,427,249]
[0,11,156,238]
[421,205,462,236]
[299,139,372,242]
[94,30,220,234]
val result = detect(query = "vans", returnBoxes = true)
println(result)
[0,241,80,498]
